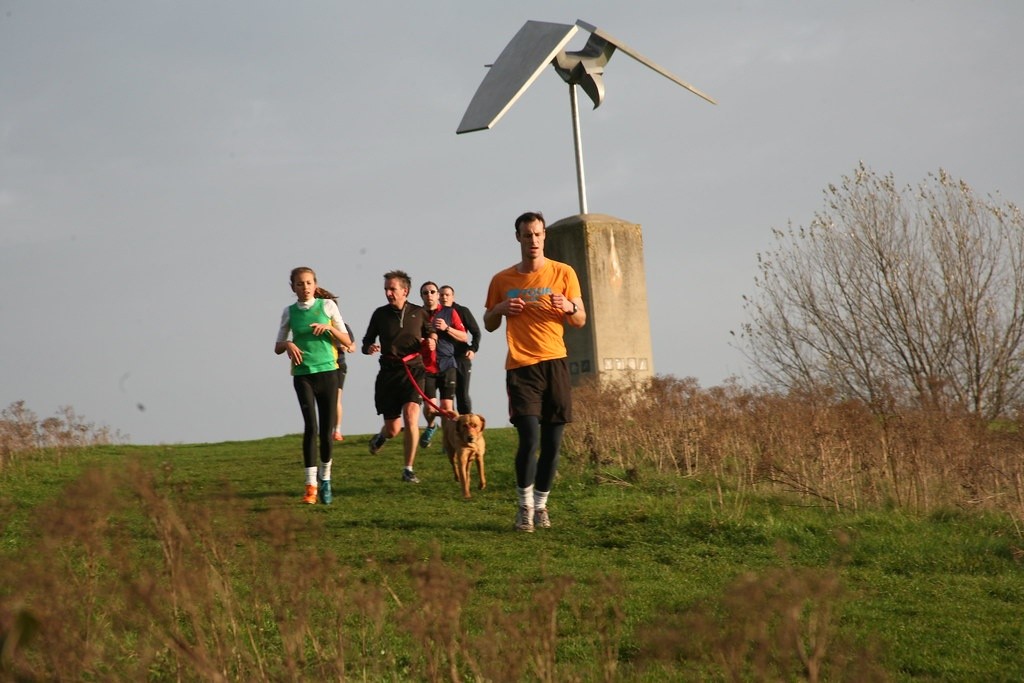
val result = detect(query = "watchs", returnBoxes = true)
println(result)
[566,303,578,315]
[444,325,449,332]
[430,337,437,343]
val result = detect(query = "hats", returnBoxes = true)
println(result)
[315,287,339,299]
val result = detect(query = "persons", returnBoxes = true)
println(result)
[420,281,457,448]
[275,267,352,505]
[314,286,356,442]
[361,270,438,484]
[484,211,587,534]
[438,285,481,415]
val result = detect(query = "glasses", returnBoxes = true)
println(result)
[421,290,438,295]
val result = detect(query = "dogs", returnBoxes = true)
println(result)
[425,409,487,499]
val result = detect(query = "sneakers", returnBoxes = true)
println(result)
[317,474,339,501]
[368,433,388,455]
[332,431,343,442]
[302,484,318,504]
[515,505,535,532]
[534,509,551,529]
[420,422,442,448]
[441,446,448,455]
[402,468,421,484]
